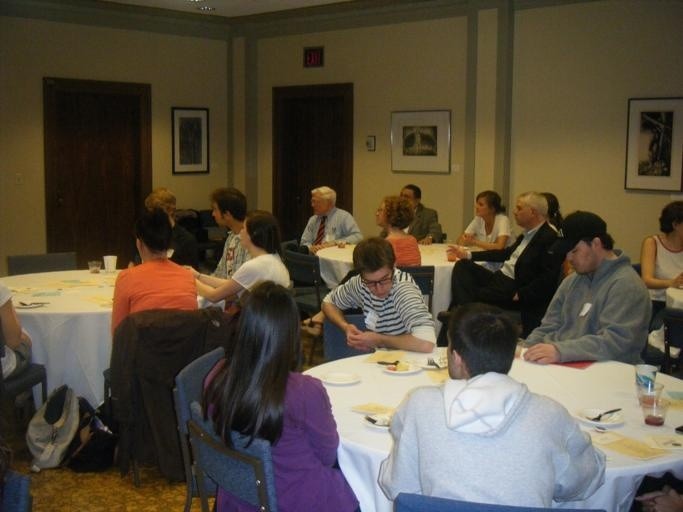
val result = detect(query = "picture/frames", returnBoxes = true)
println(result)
[622,96,682,194]
[169,105,210,176]
[388,108,452,177]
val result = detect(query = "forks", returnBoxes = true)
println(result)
[586,409,623,421]
[427,359,440,368]
[365,416,377,423]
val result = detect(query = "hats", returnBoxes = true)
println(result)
[550,211,606,254]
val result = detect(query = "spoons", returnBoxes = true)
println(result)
[19,301,50,305]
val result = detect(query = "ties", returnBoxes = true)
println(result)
[315,217,326,245]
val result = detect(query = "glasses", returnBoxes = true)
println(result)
[361,271,394,287]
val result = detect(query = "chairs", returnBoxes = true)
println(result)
[2,473,33,510]
[173,348,226,512]
[1,363,48,463]
[390,490,608,512]
[395,263,435,315]
[320,313,377,364]
[174,208,331,367]
[6,250,78,277]
[102,311,214,488]
[188,401,279,512]
[640,306,683,380]
[630,262,641,279]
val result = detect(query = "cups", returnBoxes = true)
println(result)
[636,364,671,427]
[419,234,432,249]
[88,261,102,274]
[515,338,525,358]
[446,239,459,261]
[102,254,118,273]
[336,237,345,248]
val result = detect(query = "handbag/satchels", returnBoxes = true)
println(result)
[25,384,82,468]
[68,397,114,473]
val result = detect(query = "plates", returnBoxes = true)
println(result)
[320,372,362,385]
[570,408,625,427]
[12,300,44,309]
[383,363,422,374]
[416,355,449,370]
[362,414,390,430]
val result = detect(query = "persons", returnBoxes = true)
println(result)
[0,281,33,422]
